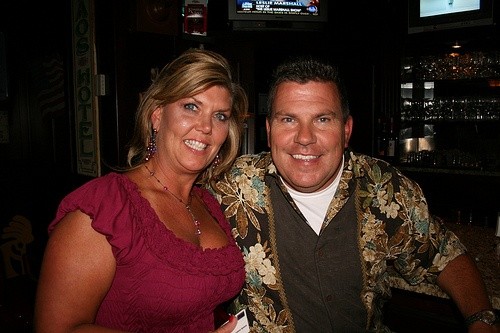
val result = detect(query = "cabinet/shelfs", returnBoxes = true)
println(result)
[395,52,500,178]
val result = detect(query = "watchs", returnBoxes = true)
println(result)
[465,309,496,326]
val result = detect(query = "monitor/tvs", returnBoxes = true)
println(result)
[228,0,330,23]
[408,0,494,34]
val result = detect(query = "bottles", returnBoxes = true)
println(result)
[385,117,399,158]
[378,120,389,153]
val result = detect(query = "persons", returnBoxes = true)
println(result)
[35,48,249,333]
[194,60,500,333]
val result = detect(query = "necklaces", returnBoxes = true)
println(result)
[142,159,201,236]
